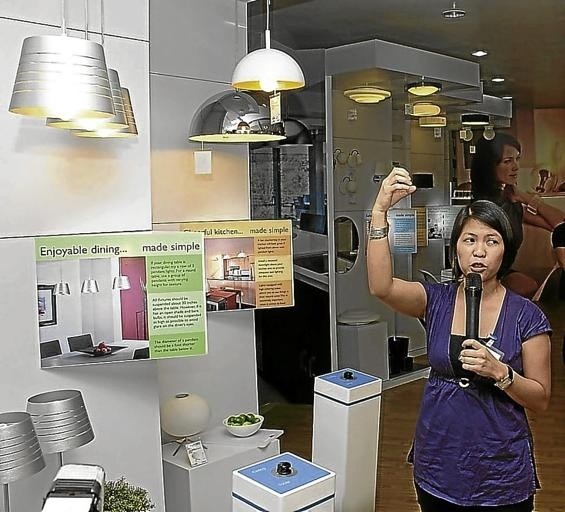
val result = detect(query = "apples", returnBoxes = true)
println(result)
[95,343,111,352]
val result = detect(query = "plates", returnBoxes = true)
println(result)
[79,346,128,356]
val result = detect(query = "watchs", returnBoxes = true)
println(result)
[495,364,514,391]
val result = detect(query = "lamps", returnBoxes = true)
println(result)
[80,261,99,293]
[47,0,129,130]
[460,113,489,125]
[343,82,392,103]
[52,261,70,296]
[461,126,473,141]
[113,257,131,290]
[189,0,287,143]
[339,172,357,204]
[420,117,447,127]
[404,75,441,95]
[26,389,96,467]
[223,240,231,259]
[482,124,494,140]
[404,101,440,116]
[74,0,138,136]
[333,148,346,174]
[0,412,47,512]
[233,0,305,93]
[236,239,247,258]
[160,392,210,456]
[244,6,312,147]
[372,161,391,183]
[347,145,361,169]
[9,0,115,120]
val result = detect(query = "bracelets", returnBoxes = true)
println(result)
[370,222,390,239]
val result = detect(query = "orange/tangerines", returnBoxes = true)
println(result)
[228,414,261,427]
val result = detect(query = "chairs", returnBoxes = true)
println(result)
[67,333,93,353]
[40,339,62,358]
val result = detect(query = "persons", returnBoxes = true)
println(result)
[469,133,539,296]
[512,183,565,272]
[366,166,552,512]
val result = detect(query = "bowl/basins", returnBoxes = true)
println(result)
[223,415,264,437]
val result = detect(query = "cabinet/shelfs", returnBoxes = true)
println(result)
[161,427,281,512]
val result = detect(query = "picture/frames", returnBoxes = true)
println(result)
[37,284,57,327]
[185,440,208,467]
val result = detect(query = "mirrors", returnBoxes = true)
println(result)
[334,215,360,275]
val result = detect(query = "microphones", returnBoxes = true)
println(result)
[466,273,483,381]
